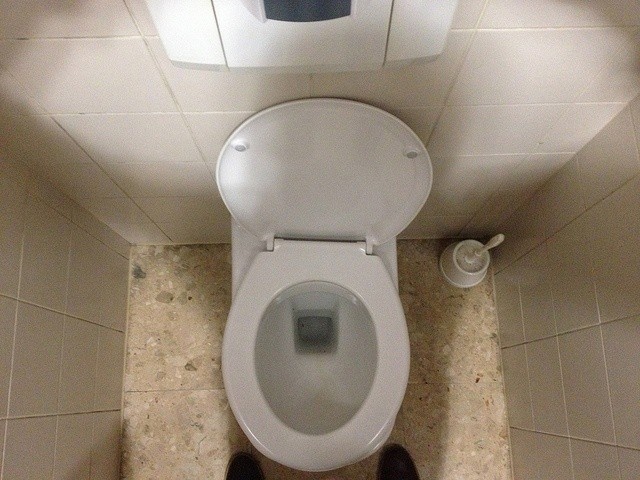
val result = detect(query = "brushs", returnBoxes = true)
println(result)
[456,233,505,273]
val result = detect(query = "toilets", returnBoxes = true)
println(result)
[216,99,435,473]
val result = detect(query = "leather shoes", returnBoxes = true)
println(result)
[225,453,264,480]
[377,444,420,480]
